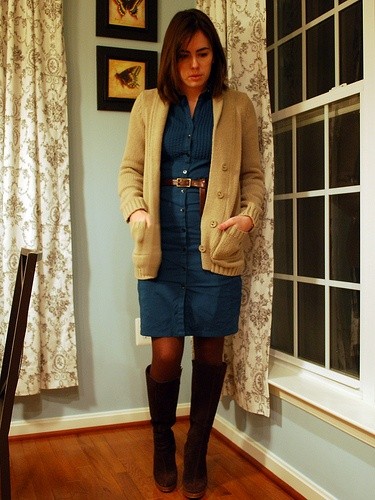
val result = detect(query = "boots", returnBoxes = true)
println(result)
[145,363,182,490]
[180,360,227,497]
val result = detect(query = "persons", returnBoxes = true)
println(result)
[118,6,269,500]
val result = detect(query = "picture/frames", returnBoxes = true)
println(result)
[96,0,158,43]
[96,45,158,113]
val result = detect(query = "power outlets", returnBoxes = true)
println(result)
[136,318,152,346]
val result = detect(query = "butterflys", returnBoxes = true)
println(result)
[114,66,141,89]
[113,0,144,21]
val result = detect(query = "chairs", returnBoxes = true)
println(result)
[0,247,42,500]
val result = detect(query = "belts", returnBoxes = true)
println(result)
[159,177,208,188]
